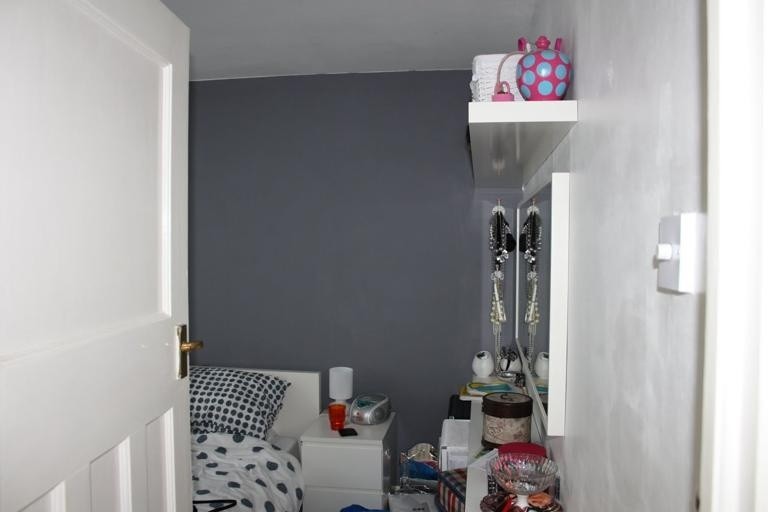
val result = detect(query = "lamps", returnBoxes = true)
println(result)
[328,367,354,424]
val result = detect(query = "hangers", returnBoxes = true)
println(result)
[193,500,237,512]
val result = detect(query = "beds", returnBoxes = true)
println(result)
[189,364,322,512]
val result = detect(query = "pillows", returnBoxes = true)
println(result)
[189,366,291,441]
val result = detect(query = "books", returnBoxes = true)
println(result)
[458,380,514,401]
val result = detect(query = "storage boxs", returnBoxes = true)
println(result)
[437,468,467,512]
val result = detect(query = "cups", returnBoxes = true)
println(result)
[329,404,346,431]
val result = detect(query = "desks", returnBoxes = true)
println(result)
[439,375,542,512]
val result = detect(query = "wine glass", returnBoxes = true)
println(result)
[489,453,558,512]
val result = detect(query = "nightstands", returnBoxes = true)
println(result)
[300,408,400,512]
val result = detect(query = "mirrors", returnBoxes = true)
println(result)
[513,171,569,437]
